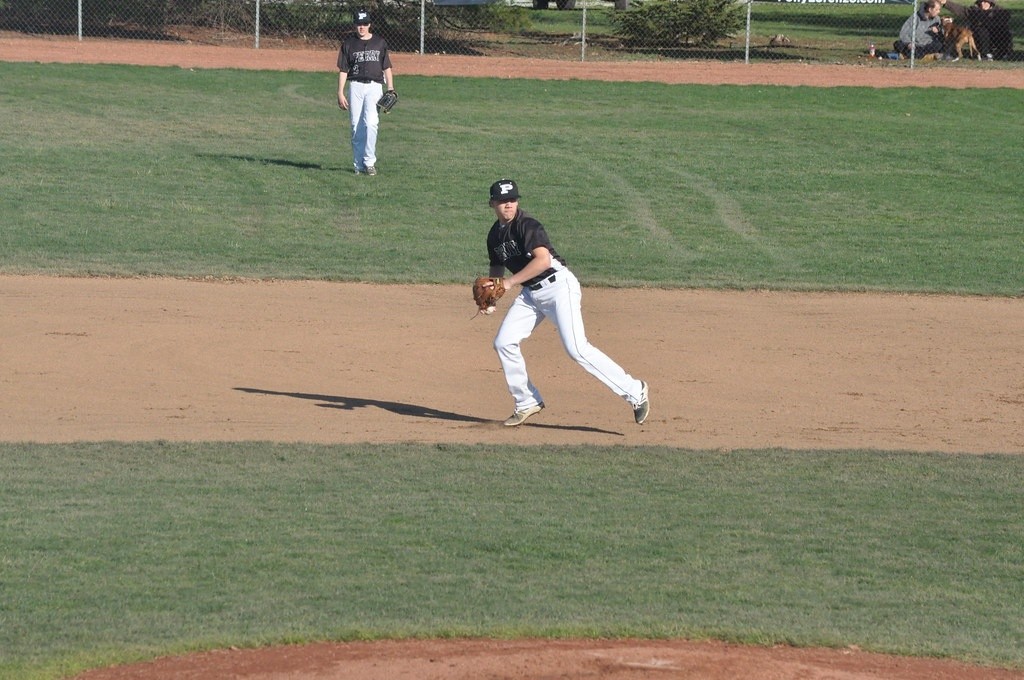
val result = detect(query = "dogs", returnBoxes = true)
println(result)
[940,15,981,62]
[921,53,941,62]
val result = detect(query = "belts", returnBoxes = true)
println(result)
[530,275,555,290]
[351,79,382,83]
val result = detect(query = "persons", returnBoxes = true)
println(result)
[337,9,399,177]
[479,180,650,427]
[893,0,1013,62]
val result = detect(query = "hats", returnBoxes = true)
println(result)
[489,180,519,200]
[354,9,370,23]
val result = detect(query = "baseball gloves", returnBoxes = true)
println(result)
[376,90,398,115]
[473,275,506,310]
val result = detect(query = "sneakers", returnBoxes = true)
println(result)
[632,380,650,423]
[355,166,365,174]
[503,402,545,425]
[363,164,376,175]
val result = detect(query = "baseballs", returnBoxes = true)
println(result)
[484,306,495,314]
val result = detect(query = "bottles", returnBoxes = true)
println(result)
[870,45,875,57]
